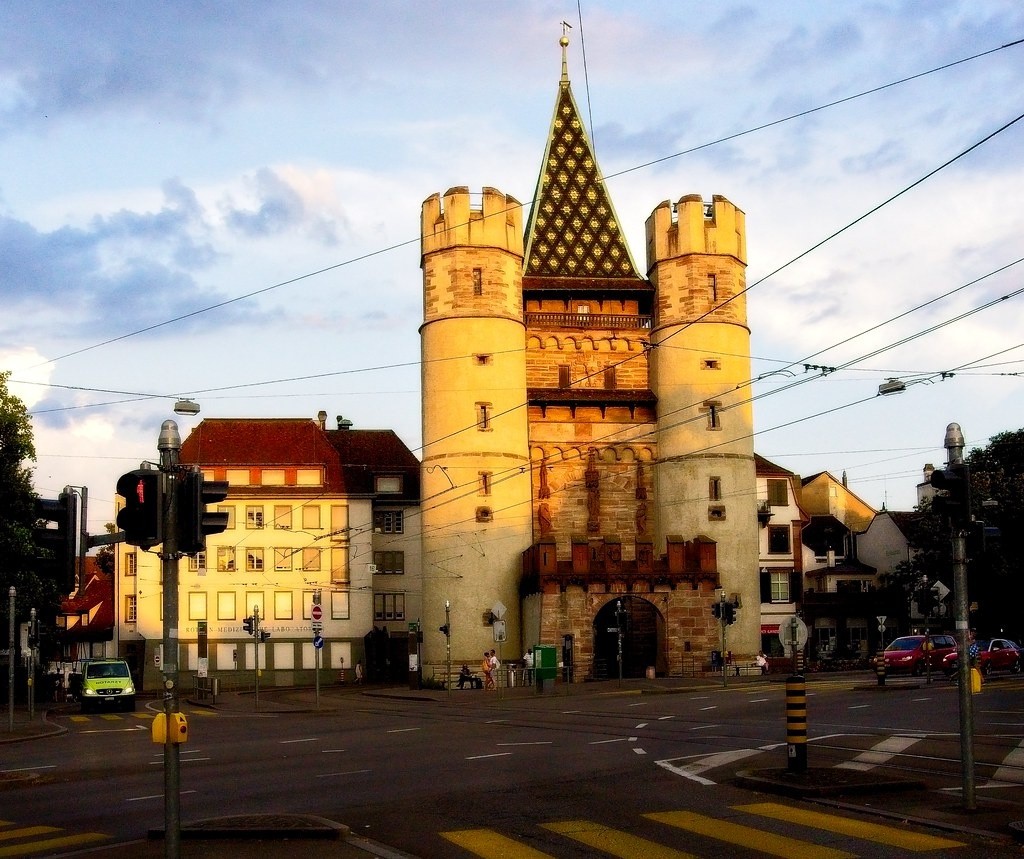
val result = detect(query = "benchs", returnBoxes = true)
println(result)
[733,657,762,677]
[440,672,478,686]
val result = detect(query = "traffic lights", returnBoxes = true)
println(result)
[243,616,254,635]
[261,631,270,643]
[36,619,47,643]
[711,603,722,618]
[725,602,737,624]
[116,469,164,548]
[439,625,447,633]
[928,464,970,519]
[967,519,999,553]
[176,472,231,555]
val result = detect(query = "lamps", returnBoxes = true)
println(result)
[878,379,906,396]
[174,400,200,416]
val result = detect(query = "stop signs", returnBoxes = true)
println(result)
[312,607,322,621]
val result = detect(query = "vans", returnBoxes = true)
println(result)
[79,657,137,712]
[874,634,957,677]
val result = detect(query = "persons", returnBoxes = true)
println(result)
[755,650,767,675]
[54,668,65,702]
[460,663,474,690]
[521,649,535,686]
[968,629,987,684]
[354,660,362,685]
[68,668,79,703]
[484,649,497,691]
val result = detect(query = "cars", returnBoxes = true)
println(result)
[943,639,1024,676]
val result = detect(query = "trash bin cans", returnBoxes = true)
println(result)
[506,663,518,687]
